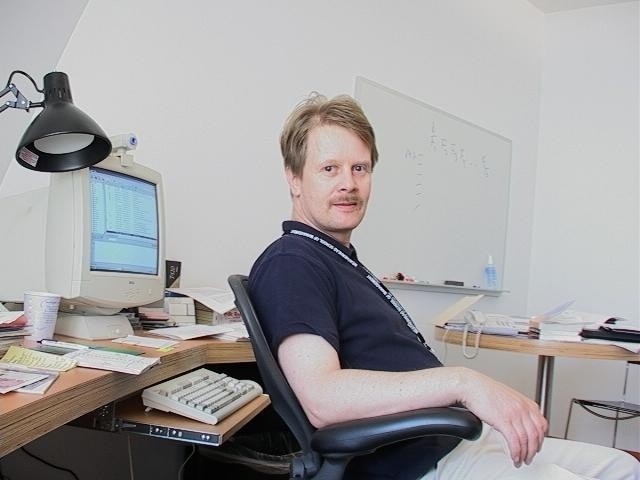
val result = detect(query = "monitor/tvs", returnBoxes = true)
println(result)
[44,152,167,340]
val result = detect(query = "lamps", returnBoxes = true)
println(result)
[0,70,113,172]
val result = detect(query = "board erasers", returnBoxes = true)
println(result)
[445,281,464,286]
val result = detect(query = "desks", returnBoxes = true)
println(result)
[0,331,258,459]
[433,325,640,438]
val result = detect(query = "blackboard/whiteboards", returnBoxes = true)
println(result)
[353,74,512,297]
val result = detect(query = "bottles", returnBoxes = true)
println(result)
[484,255,498,290]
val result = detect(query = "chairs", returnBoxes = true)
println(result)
[229,273,483,480]
[564,359,640,448]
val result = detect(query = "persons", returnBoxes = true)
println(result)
[247,89,640,480]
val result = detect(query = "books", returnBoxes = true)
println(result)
[0,287,251,396]
[431,295,640,355]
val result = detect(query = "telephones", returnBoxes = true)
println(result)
[465,310,518,335]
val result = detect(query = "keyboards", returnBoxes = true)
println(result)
[141,368,263,426]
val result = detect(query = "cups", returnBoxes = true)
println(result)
[22,289,62,344]
